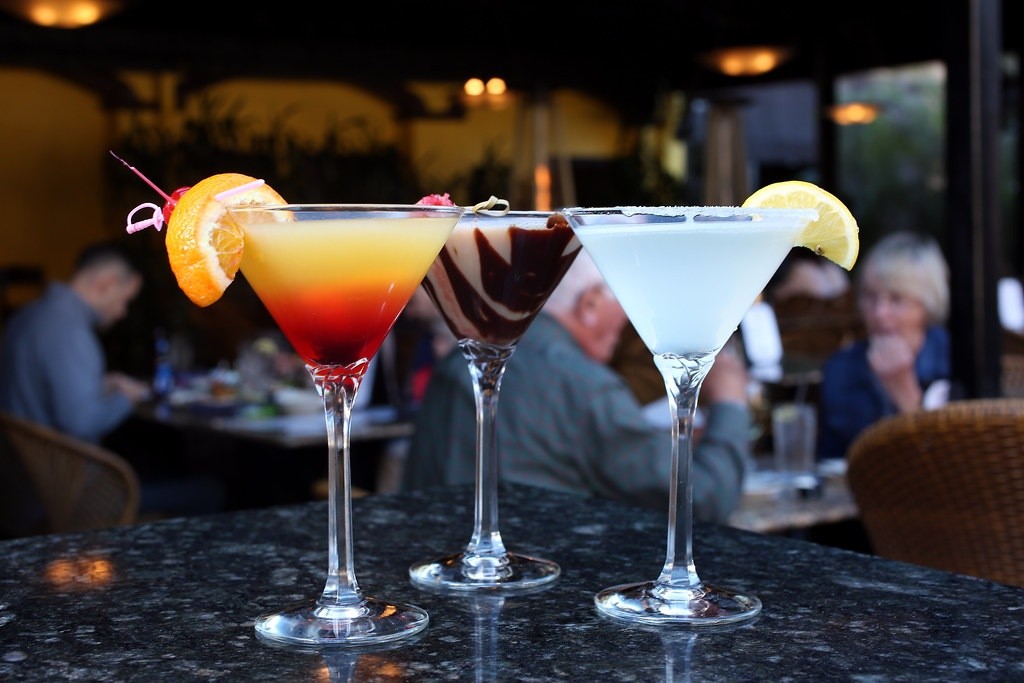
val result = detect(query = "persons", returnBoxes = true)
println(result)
[0,258,47,331]
[817,233,949,461]
[403,249,756,528]
[4,240,217,518]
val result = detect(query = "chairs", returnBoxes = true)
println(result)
[0,409,144,531]
[845,398,1024,594]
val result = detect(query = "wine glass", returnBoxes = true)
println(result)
[405,206,581,590]
[228,205,431,649]
[563,204,823,629]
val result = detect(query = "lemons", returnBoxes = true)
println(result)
[740,181,860,271]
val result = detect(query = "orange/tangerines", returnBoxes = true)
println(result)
[166,171,296,309]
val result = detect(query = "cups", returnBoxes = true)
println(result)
[772,403,817,474]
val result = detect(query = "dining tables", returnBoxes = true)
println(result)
[107,365,429,511]
[709,454,868,537]
[0,474,1024,683]
